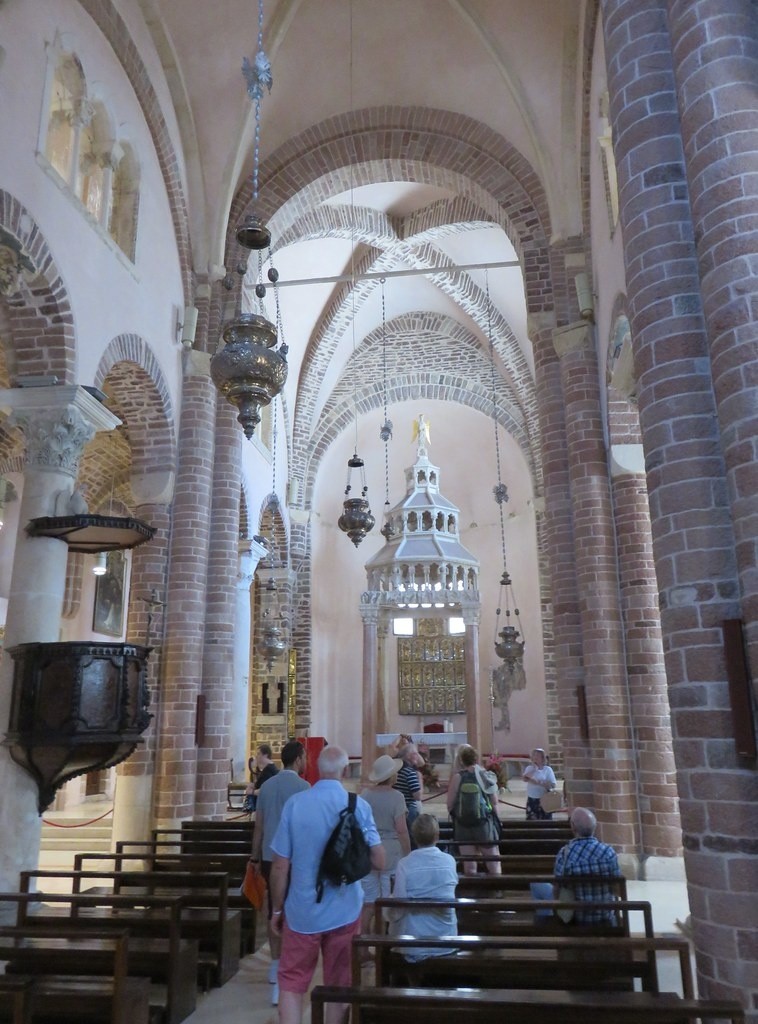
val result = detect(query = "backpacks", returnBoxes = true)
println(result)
[453,768,492,826]
[316,792,372,887]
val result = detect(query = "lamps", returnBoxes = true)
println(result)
[484,267,529,678]
[176,306,199,348]
[575,273,595,316]
[379,278,396,541]
[208,1,290,438]
[92,550,107,575]
[258,266,286,676]
[339,167,375,545]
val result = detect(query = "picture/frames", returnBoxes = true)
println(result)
[92,547,126,637]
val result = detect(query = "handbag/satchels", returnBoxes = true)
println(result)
[416,754,426,768]
[540,785,562,814]
[242,864,267,911]
[242,794,256,813]
[553,844,576,924]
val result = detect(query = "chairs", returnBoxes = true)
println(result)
[227,758,247,811]
[423,723,445,763]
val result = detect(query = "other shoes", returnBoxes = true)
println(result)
[272,988,279,1005]
[359,951,376,968]
[269,969,278,984]
[494,889,503,898]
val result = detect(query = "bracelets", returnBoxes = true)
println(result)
[250,857,259,863]
[252,789,254,795]
[273,910,283,914]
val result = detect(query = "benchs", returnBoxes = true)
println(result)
[346,756,362,777]
[482,753,532,780]
[309,817,746,1024]
[0,818,278,1024]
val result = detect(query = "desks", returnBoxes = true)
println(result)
[375,732,468,748]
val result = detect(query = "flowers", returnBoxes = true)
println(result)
[416,745,443,793]
[484,754,513,797]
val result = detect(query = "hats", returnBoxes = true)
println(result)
[368,755,403,782]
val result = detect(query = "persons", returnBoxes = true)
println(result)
[245,744,279,796]
[356,755,411,933]
[382,812,460,989]
[386,734,425,831]
[269,745,385,1024]
[447,745,503,898]
[523,749,556,821]
[530,806,620,921]
[249,741,312,982]
